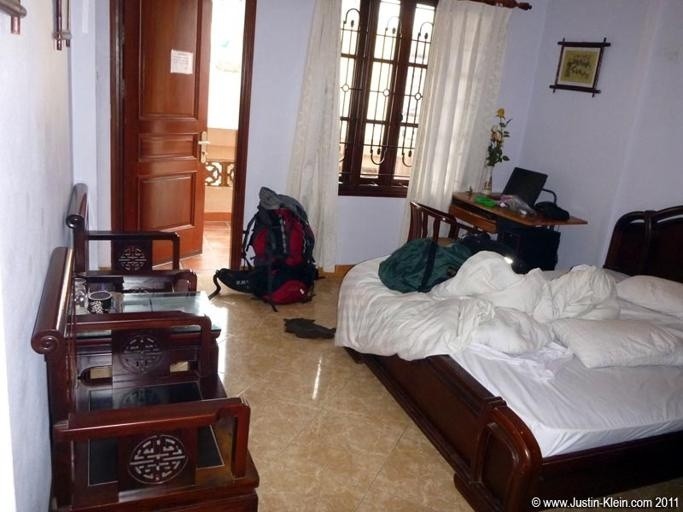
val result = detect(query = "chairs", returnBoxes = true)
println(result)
[66,183,197,295]
[407,200,482,242]
[30,245,260,512]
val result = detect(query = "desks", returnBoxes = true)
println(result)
[448,191,588,274]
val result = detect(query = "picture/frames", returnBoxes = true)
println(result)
[53,0,72,51]
[549,37,611,98]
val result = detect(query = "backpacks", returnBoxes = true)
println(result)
[208,188,325,312]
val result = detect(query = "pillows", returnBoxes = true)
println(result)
[616,275,683,319]
[552,317,683,370]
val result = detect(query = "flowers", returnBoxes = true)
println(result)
[485,107,513,166]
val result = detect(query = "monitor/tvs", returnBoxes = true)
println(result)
[503,167,548,208]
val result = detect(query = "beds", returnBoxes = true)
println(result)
[343,204,683,511]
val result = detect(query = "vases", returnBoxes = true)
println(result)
[480,166,495,195]
